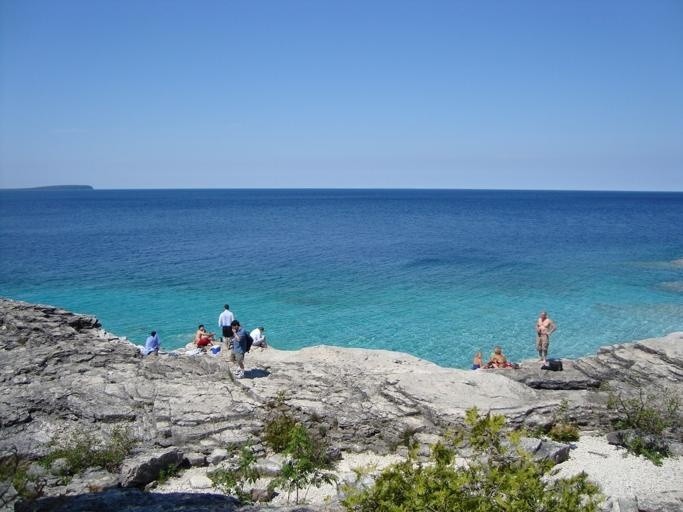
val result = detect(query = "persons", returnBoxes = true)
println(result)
[228,320,246,378]
[248,326,265,347]
[534,311,557,363]
[483,347,506,369]
[470,352,482,370]
[217,303,235,350]
[144,331,159,355]
[202,329,215,341]
[193,324,215,346]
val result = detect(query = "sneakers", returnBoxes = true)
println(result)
[236,371,244,379]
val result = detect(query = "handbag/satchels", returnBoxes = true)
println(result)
[245,334,253,352]
